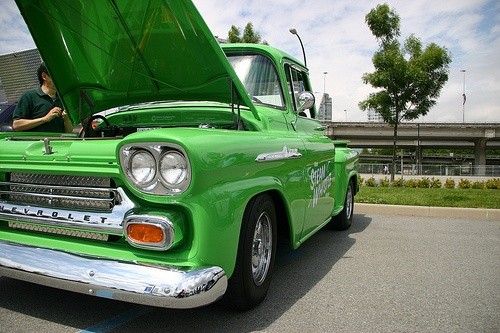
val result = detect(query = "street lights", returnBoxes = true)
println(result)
[290,26,308,68]
[460,69,467,123]
[322,71,328,121]
[344,109,348,121]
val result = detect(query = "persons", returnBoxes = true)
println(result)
[11,63,74,134]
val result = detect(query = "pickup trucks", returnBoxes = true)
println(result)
[0,1,360,314]
